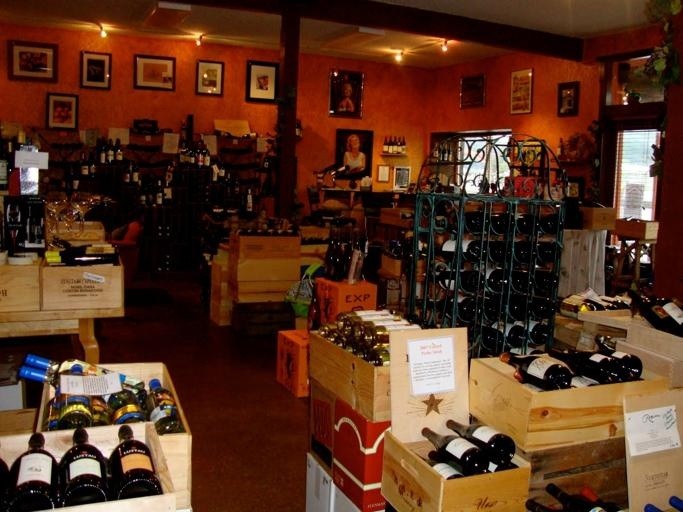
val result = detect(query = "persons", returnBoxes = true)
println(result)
[342,134,365,172]
[337,83,355,113]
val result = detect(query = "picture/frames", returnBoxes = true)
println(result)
[328,67,374,178]
[458,68,580,117]
[375,165,411,193]
[4,38,281,130]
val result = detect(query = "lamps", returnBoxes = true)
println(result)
[194,33,206,47]
[94,21,107,38]
[394,52,403,63]
[441,39,448,52]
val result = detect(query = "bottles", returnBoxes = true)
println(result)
[620,283,683,336]
[556,137,564,161]
[381,135,405,154]
[315,307,440,365]
[237,226,299,237]
[431,209,643,391]
[213,130,232,138]
[124,144,255,211]
[421,419,683,512]
[320,162,350,176]
[0,355,181,512]
[0,121,124,266]
[262,151,269,168]
[179,120,186,139]
[323,234,429,283]
[432,141,452,160]
[240,132,257,139]
